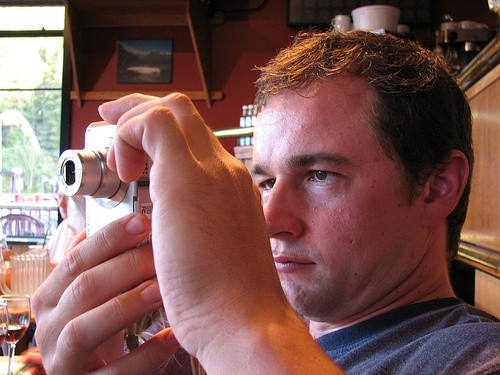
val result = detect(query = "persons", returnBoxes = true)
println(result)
[30,28,500,375]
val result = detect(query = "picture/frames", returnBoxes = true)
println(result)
[117,38,175,84]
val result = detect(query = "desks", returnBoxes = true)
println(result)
[6,236,44,253]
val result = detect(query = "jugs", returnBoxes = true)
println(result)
[0,248,50,319]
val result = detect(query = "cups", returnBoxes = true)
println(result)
[440,21,487,30]
[0,298,8,347]
[330,4,410,34]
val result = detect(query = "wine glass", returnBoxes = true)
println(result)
[1,294,31,375]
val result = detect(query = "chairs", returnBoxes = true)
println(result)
[0,214,44,236]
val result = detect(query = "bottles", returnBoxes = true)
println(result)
[240,104,257,128]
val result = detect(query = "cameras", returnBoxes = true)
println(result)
[56,121,155,247]
